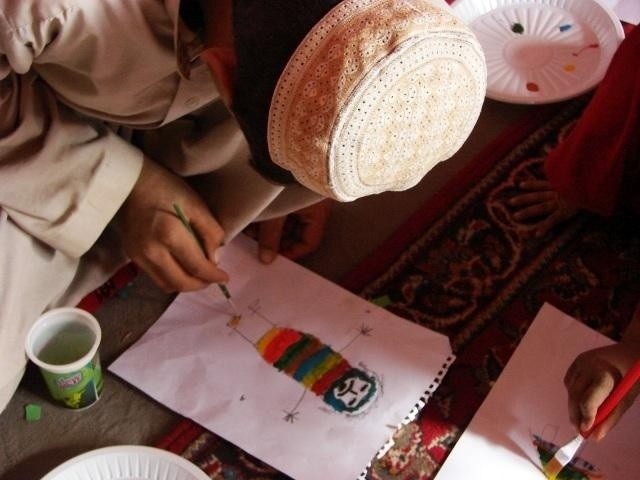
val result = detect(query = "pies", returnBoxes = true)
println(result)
[173,203,238,314]
[542,359,640,480]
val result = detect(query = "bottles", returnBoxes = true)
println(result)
[267,0,488,205]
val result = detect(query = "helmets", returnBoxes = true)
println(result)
[450,0,625,106]
[40,445,213,480]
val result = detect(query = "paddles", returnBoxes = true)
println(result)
[153,96,640,480]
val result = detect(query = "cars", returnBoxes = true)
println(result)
[23,305,106,413]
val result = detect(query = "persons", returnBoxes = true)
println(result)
[0,0,490,417]
[563,302,640,443]
[507,23,640,237]
